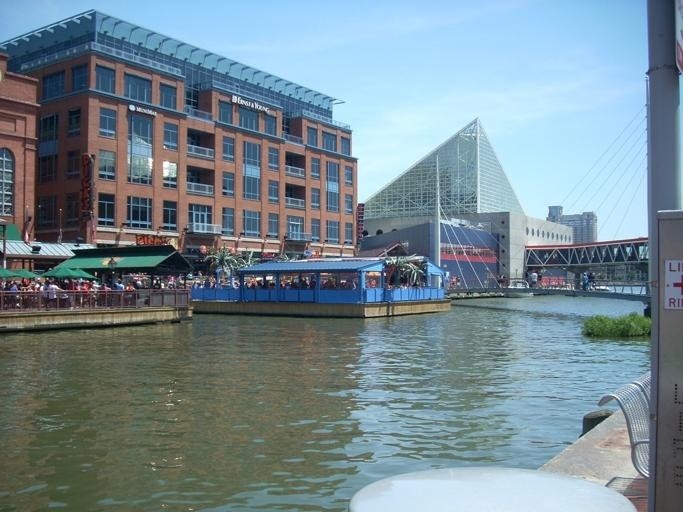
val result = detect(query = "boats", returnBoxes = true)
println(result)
[502,278,534,298]
[595,285,612,291]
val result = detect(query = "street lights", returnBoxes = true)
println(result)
[260,234,271,256]
[114,222,128,247]
[178,228,189,252]
[276,233,359,258]
[58,208,63,241]
[233,232,246,252]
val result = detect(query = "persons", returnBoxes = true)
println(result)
[497,273,510,289]
[525,268,543,288]
[582,272,596,293]
[448,275,462,288]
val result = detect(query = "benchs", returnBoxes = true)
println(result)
[595,369,652,480]
